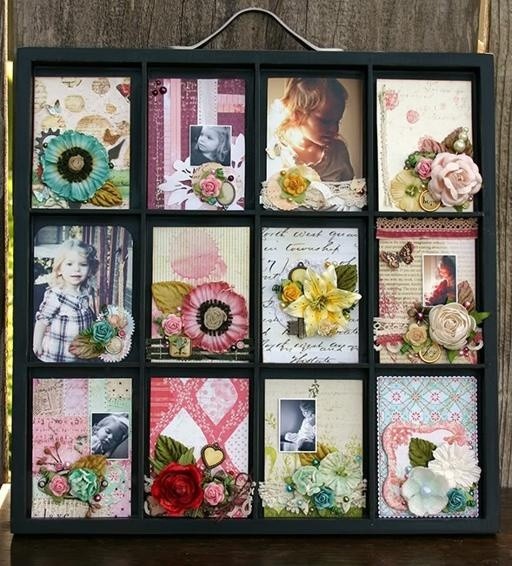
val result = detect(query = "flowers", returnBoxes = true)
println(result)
[400,280,493,364]
[191,161,225,206]
[258,441,367,516]
[151,280,249,354]
[277,165,312,204]
[398,437,483,517]
[39,130,123,207]
[145,434,256,519]
[36,441,109,516]
[277,260,363,337]
[68,304,136,364]
[390,126,483,213]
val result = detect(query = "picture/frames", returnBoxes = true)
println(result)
[10,47,500,536]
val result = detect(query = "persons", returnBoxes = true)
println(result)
[190,126,231,166]
[434,255,456,305]
[32,238,98,364]
[91,414,129,455]
[266,76,355,182]
[283,400,316,451]
